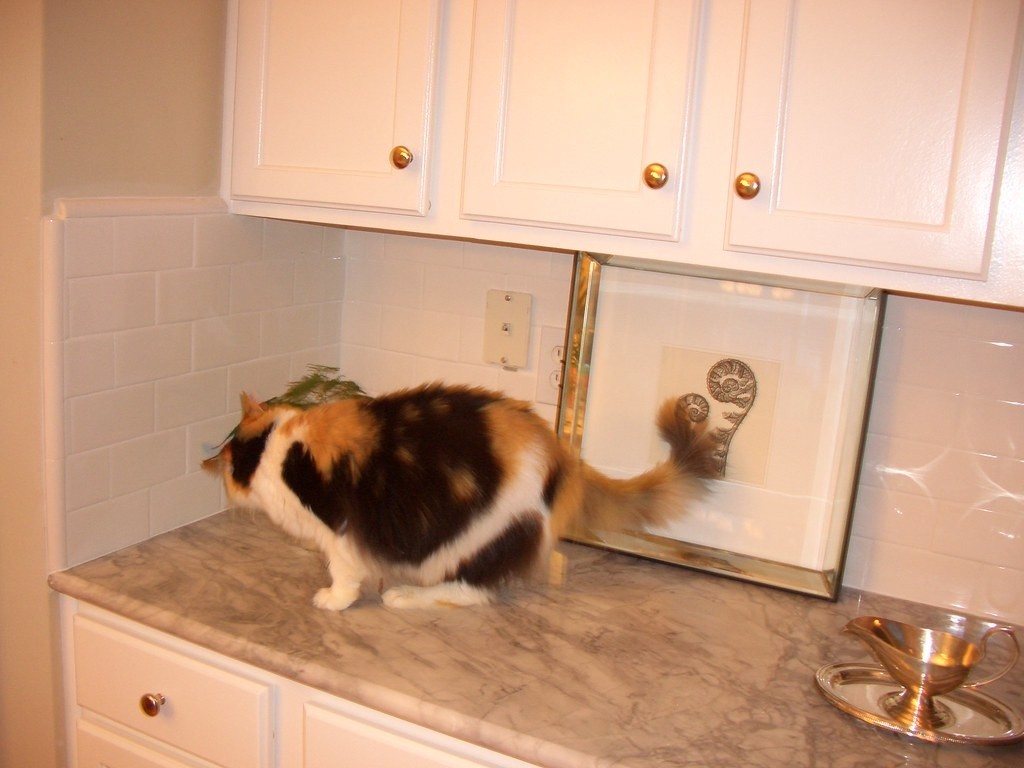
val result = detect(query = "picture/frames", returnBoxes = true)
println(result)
[551,252,887,606]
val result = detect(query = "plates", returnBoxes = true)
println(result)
[814,662,1024,748]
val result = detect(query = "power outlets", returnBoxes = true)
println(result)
[533,324,565,405]
[480,290,531,369]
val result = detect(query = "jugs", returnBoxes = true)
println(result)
[839,616,1020,729]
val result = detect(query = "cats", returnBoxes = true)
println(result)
[202,378,729,613]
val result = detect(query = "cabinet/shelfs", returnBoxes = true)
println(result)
[67,604,544,768]
[438,0,1024,321]
[221,0,449,243]
